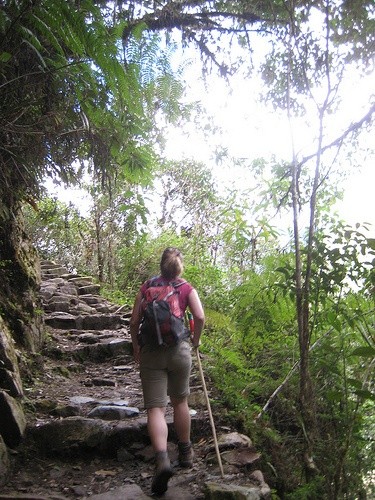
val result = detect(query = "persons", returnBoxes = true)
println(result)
[130,248,205,496]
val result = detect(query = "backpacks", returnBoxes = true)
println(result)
[139,278,188,355]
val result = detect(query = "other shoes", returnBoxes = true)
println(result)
[177,442,195,467]
[151,459,172,494]
